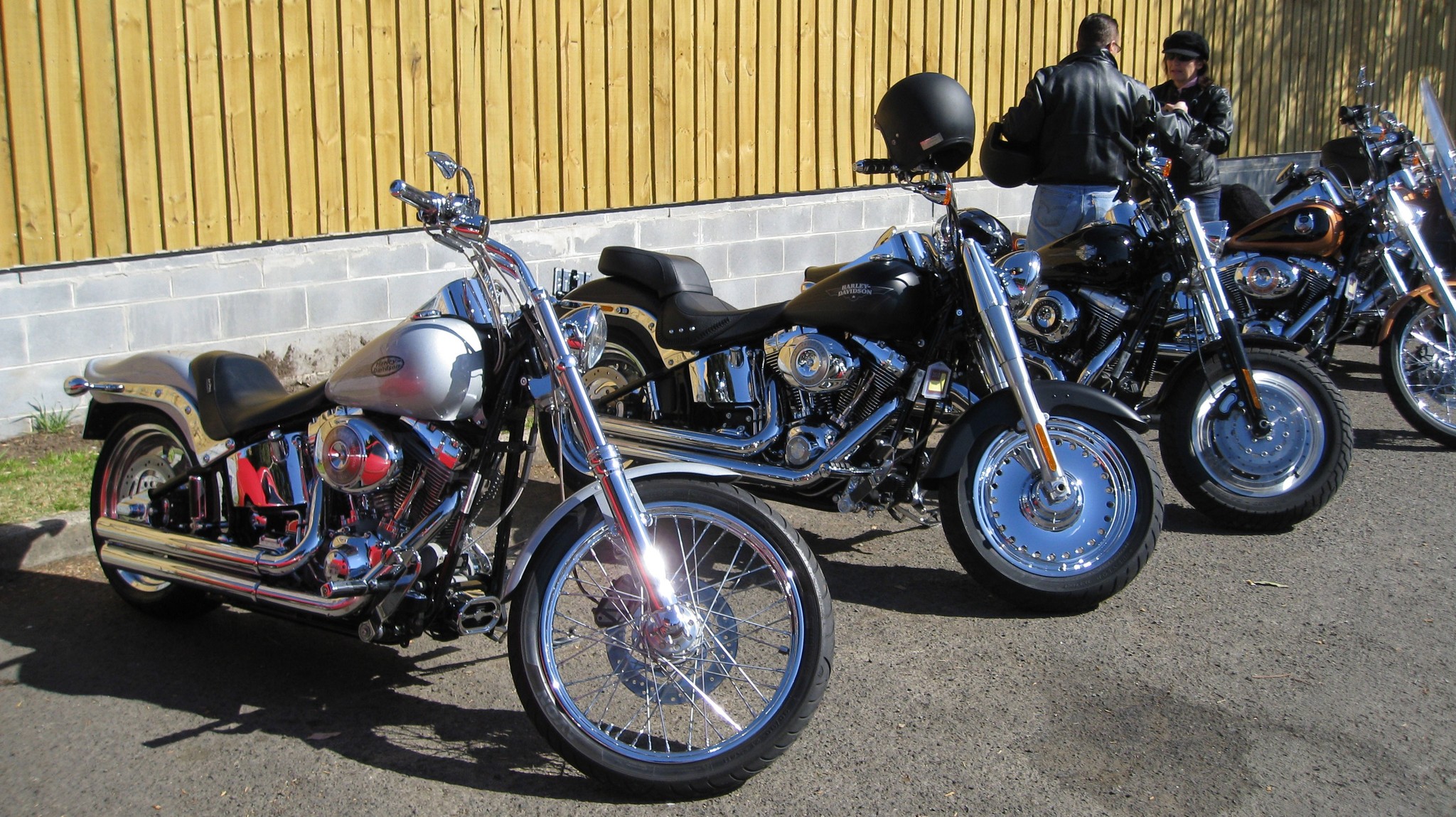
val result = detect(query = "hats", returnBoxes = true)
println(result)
[1162,31,1210,60]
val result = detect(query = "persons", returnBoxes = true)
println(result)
[980,14,1193,253]
[1149,31,1236,222]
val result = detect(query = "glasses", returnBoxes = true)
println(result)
[1165,54,1199,62]
[1107,42,1122,53]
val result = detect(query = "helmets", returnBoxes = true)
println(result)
[873,72,976,173]
[979,122,1034,188]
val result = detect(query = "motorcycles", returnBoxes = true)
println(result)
[1156,65,1456,450]
[63,151,838,800]
[529,70,1167,613]
[931,129,1357,527]
